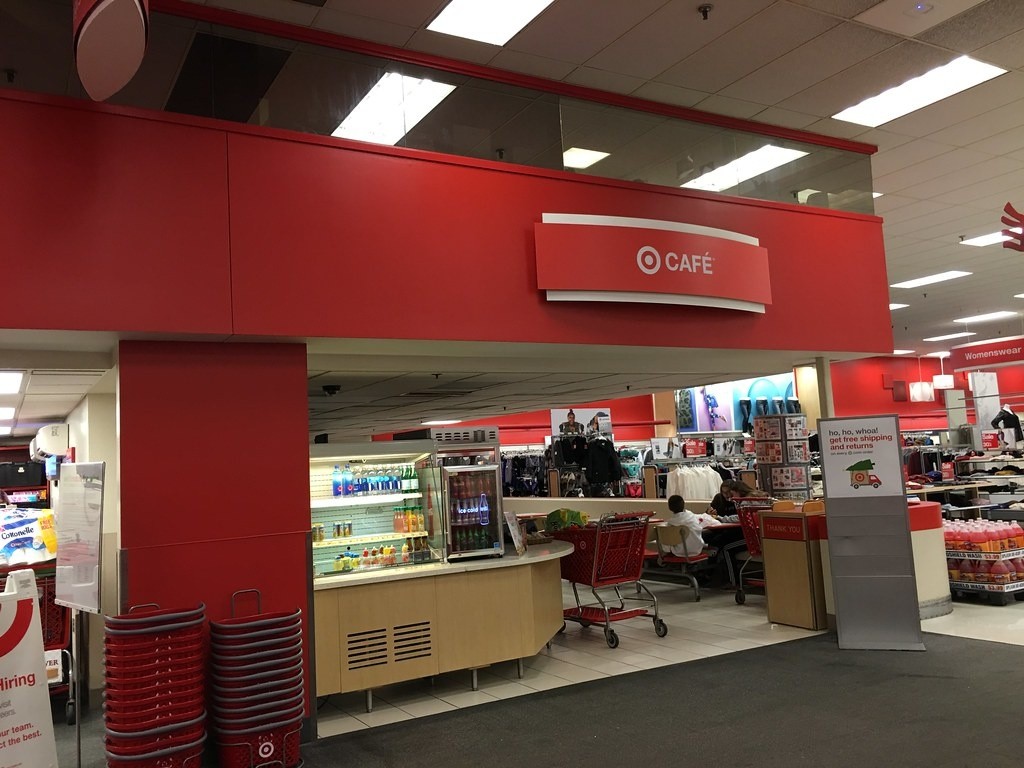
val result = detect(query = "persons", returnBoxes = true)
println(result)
[756,397,768,415]
[661,495,721,557]
[587,412,608,435]
[997,429,1008,446]
[701,479,769,593]
[991,404,1024,450]
[772,397,785,415]
[559,409,584,435]
[740,397,752,432]
[787,397,800,413]
[700,386,727,430]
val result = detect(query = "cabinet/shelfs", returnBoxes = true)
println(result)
[906,451,1024,522]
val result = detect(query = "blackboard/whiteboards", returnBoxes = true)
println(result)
[53,462,106,614]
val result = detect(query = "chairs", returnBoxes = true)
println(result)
[653,524,708,565]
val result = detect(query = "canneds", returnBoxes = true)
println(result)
[312,521,351,542]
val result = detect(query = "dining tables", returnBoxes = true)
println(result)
[516,512,740,529]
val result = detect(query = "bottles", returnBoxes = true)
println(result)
[453,526,495,552]
[942,516,1024,551]
[401,542,409,563]
[451,473,494,497]
[479,493,489,525]
[333,464,418,498]
[427,484,435,541]
[371,546,378,566]
[414,537,422,562]
[452,498,487,522]
[393,505,424,533]
[947,557,1024,584]
[407,538,413,562]
[379,544,397,564]
[363,547,370,568]
[423,536,430,560]
[334,551,360,569]
[38,508,57,554]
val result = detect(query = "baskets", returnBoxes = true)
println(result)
[102,591,305,768]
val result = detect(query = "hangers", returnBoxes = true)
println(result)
[498,430,935,481]
